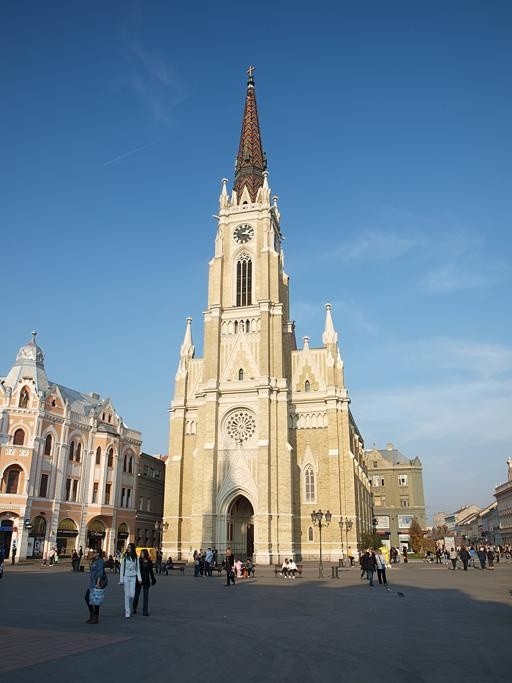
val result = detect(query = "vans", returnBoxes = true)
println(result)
[119,546,157,567]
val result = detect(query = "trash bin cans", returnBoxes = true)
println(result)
[339,559,344,567]
[331,566,339,578]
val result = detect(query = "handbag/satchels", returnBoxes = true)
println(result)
[377,554,386,569]
[229,572,235,579]
[88,588,104,607]
[96,566,108,588]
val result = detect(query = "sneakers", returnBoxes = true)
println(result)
[124,610,150,619]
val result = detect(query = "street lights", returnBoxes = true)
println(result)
[310,508,332,577]
[338,515,345,556]
[153,517,170,551]
[344,515,353,557]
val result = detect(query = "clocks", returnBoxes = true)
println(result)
[231,222,255,245]
[274,232,279,252]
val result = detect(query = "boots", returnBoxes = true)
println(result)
[86,614,100,624]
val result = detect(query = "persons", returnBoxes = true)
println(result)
[41,540,173,624]
[11,543,17,565]
[286,559,298,579]
[193,545,218,577]
[279,558,289,579]
[244,558,255,578]
[345,542,512,588]
[0,541,7,579]
[222,547,235,586]
[235,556,243,578]
